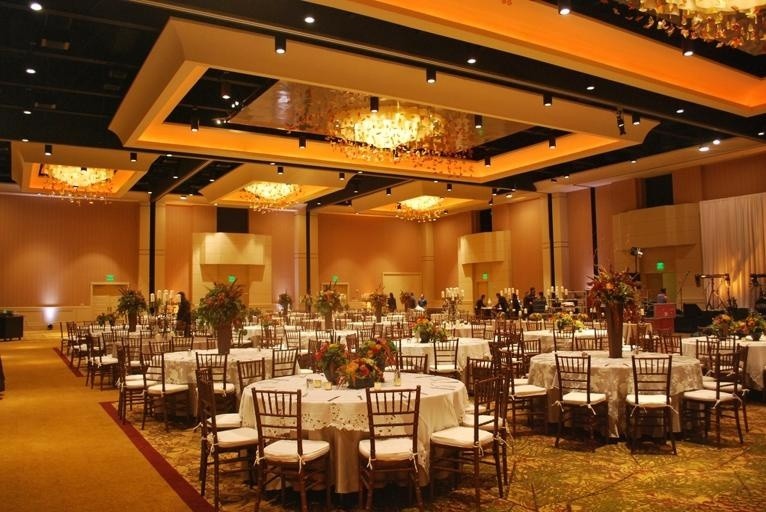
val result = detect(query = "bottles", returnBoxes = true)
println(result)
[394,368,401,386]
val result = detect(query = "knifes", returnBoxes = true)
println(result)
[327,395,341,401]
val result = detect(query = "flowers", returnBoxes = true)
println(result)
[117,283,149,316]
[277,281,417,301]
[587,263,645,308]
[197,278,247,332]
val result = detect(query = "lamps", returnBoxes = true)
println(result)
[631,247,644,259]
[681,40,694,58]
[557,0,572,16]
[36,35,643,226]
[47,324,52,329]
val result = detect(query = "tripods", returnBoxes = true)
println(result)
[706,278,727,310]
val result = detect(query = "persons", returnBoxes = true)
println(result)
[656,287,668,304]
[178,292,191,336]
[388,292,396,312]
[418,294,428,308]
[475,294,486,318]
[496,288,547,317]
[411,291,417,307]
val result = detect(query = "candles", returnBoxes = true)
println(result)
[440,284,569,300]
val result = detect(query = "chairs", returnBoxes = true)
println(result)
[428,368,512,509]
[247,383,334,509]
[624,353,677,452]
[684,348,745,450]
[193,368,260,506]
[56,297,766,432]
[550,351,612,452]
[359,385,427,510]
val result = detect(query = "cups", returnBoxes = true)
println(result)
[374,382,382,391]
[307,377,332,391]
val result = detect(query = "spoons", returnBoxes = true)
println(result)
[356,391,364,402]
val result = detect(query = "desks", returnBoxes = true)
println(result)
[1,312,23,341]
